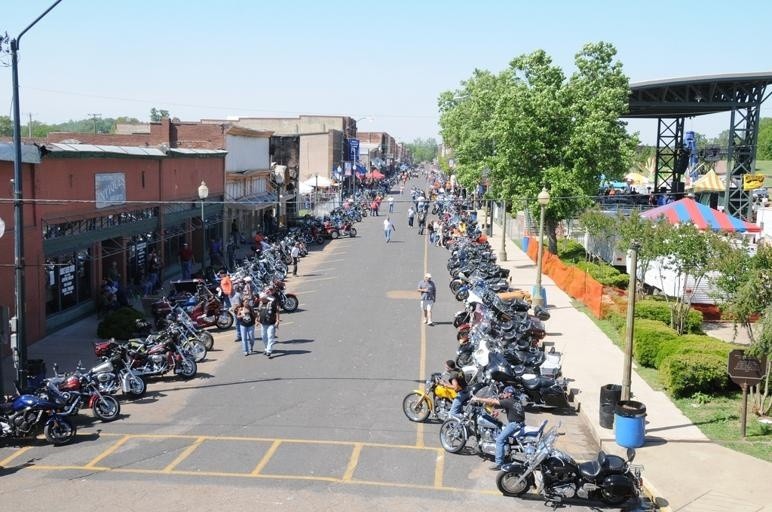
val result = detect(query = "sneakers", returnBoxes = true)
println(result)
[234,337,242,342]
[244,350,252,356]
[488,464,502,470]
[264,351,270,356]
[424,320,432,325]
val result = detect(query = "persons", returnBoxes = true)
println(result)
[438,360,469,417]
[602,186,640,196]
[98,208,301,359]
[418,273,437,327]
[476,385,525,471]
[305,195,310,209]
[327,166,483,247]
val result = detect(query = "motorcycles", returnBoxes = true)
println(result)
[214,199,370,313]
[496,421,643,511]
[403,293,568,462]
[96,286,233,378]
[0,347,145,446]
[430,208,510,301]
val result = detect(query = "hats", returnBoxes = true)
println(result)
[423,273,432,278]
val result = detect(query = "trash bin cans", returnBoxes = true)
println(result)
[27,359,47,391]
[600,384,647,448]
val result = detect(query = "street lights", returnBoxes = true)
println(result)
[198,181,209,275]
[275,174,282,233]
[337,165,357,206]
[532,187,550,309]
